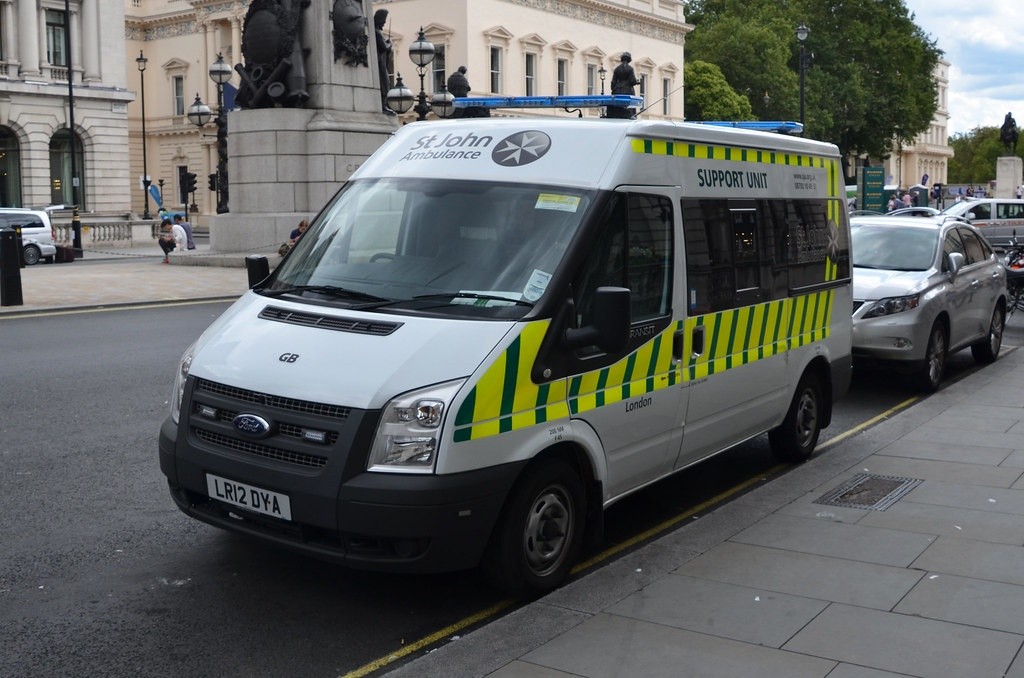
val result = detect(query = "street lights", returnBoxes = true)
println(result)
[793,20,811,139]
[385,25,456,122]
[187,52,232,213]
[597,62,607,118]
[135,49,153,220]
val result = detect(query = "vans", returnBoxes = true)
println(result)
[930,196,1024,248]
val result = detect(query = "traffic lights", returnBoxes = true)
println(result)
[179,165,197,204]
[208,173,215,191]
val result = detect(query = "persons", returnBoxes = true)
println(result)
[954,193,961,203]
[174,215,195,249]
[930,187,934,204]
[375,10,394,113]
[448,66,470,98]
[158,220,188,263]
[290,220,308,247]
[887,192,919,212]
[1016,187,1022,199]
[967,185,974,196]
[611,53,638,115]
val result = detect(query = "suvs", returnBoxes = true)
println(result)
[848,206,1008,396]
[0,204,80,266]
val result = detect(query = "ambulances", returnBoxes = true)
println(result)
[157,92,855,600]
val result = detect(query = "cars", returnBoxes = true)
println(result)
[884,205,941,217]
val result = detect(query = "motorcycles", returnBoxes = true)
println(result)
[999,229,1024,313]
